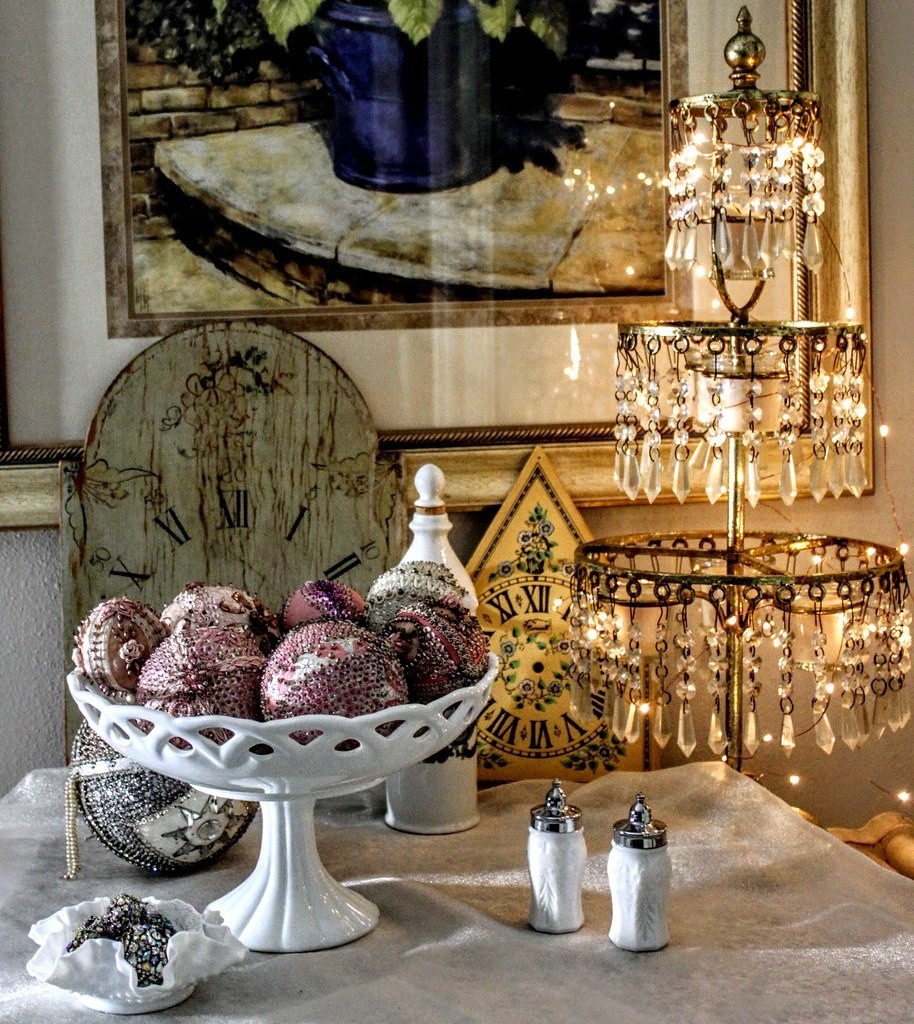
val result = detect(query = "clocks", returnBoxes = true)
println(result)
[55,320,409,764]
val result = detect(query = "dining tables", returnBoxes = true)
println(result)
[1,769,914,1024]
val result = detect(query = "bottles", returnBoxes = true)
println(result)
[365,462,485,838]
[528,777,593,933]
[607,791,675,955]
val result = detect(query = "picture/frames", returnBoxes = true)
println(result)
[0,0,874,533]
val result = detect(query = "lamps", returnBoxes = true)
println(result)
[565,8,914,772]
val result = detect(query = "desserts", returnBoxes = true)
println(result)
[75,562,488,756]
